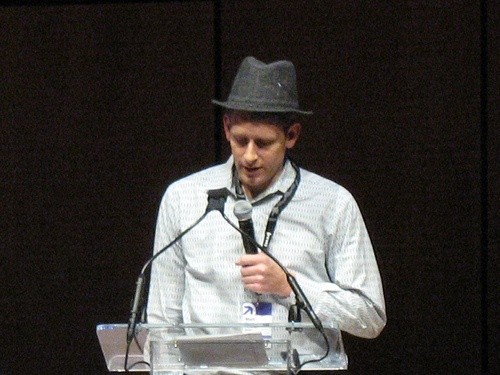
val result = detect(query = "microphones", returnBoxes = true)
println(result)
[126,188,324,344]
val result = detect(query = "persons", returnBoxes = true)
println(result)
[143,56,386,369]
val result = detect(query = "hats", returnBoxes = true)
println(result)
[211,55,314,115]
